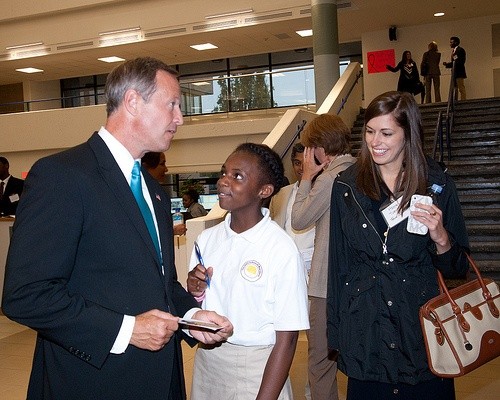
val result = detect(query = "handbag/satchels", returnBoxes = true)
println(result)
[412,80,425,96]
[419,246,500,379]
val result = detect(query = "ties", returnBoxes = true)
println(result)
[131,161,162,269]
[0,182,5,195]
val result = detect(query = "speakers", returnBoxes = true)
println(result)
[388,27,397,42]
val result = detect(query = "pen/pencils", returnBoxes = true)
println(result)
[193,240,210,290]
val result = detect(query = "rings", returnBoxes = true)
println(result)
[432,212,436,216]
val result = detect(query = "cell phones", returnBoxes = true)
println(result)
[406,195,433,235]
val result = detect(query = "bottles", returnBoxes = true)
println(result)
[425,184,443,208]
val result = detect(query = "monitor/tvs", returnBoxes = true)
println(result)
[170,197,185,213]
[198,194,219,210]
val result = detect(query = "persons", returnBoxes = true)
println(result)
[141,152,187,236]
[269,113,358,400]
[183,189,208,218]
[0,157,25,217]
[420,41,441,104]
[385,50,426,104]
[326,90,470,400]
[443,36,467,100]
[185,143,311,400]
[0,56,234,400]
